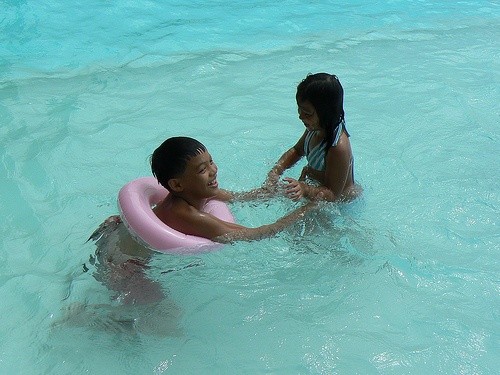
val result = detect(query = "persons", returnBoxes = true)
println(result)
[104,137,324,240]
[265,72,374,203]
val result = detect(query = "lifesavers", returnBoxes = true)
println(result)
[116,178,235,254]
